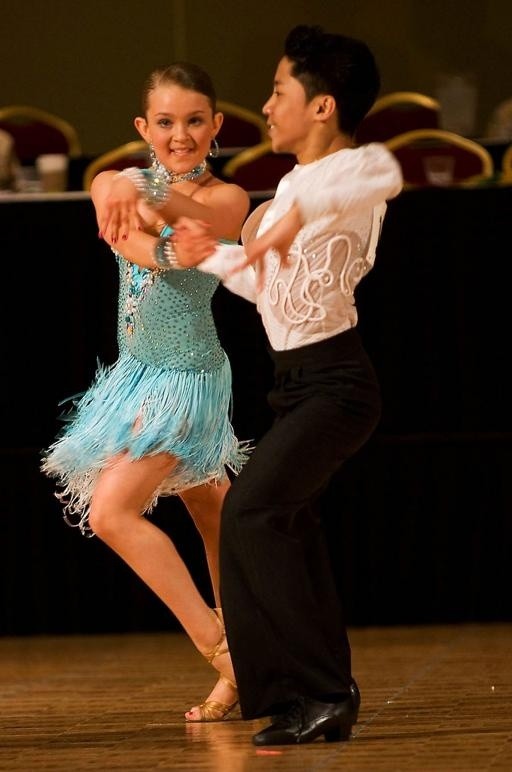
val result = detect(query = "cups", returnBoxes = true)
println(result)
[37,154,68,193]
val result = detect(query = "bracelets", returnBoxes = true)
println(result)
[152,235,185,274]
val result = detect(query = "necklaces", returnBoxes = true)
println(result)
[147,155,210,185]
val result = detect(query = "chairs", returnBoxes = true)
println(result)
[363,91,443,143]
[83,140,153,192]
[224,140,299,192]
[0,105,81,167]
[385,127,494,186]
[212,101,269,146]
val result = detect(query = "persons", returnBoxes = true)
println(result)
[41,60,250,722]
[171,24,403,747]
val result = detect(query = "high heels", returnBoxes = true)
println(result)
[186,607,250,722]
[252,680,360,746]
